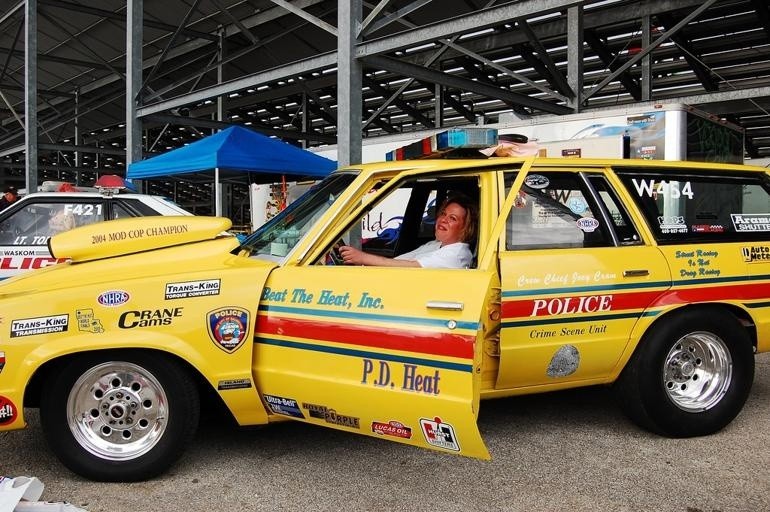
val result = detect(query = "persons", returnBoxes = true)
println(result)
[338,196,475,270]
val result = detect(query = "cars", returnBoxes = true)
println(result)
[0,175,237,298]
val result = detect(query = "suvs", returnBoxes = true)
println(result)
[1,122,770,480]
